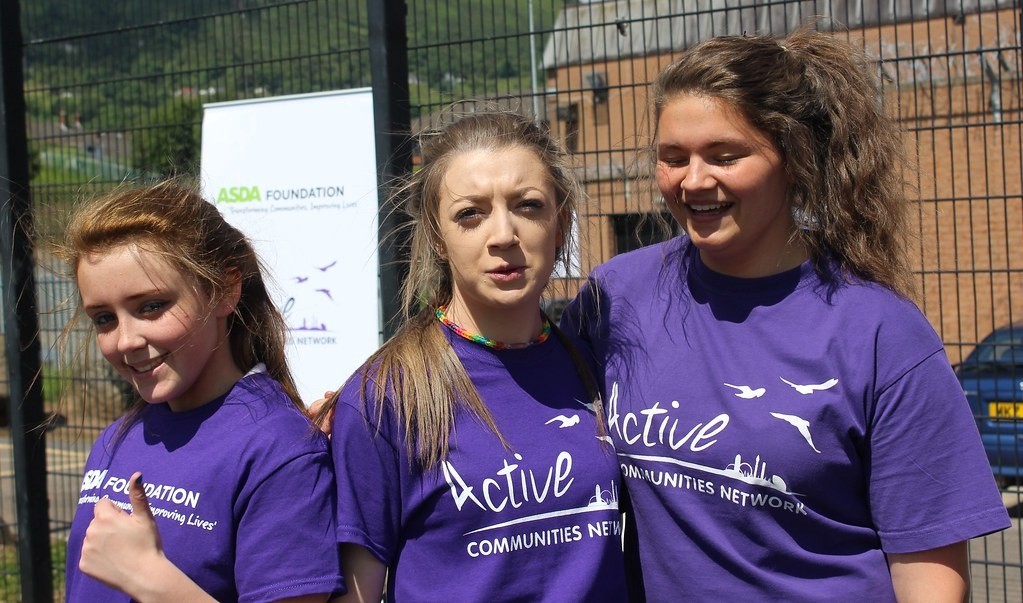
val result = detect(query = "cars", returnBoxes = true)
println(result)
[955,320,1023,490]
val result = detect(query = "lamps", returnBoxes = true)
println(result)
[585,70,609,99]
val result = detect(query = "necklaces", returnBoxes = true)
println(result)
[436,306,552,352]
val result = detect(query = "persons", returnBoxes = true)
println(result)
[306,36,1011,603]
[67,184,337,603]
